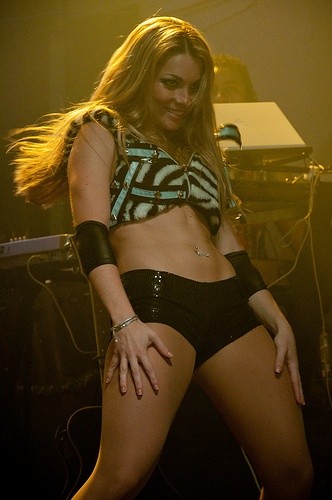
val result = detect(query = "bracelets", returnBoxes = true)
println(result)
[112,314,139,333]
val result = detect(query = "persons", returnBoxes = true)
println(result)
[208,55,304,261]
[5,15,315,500]
